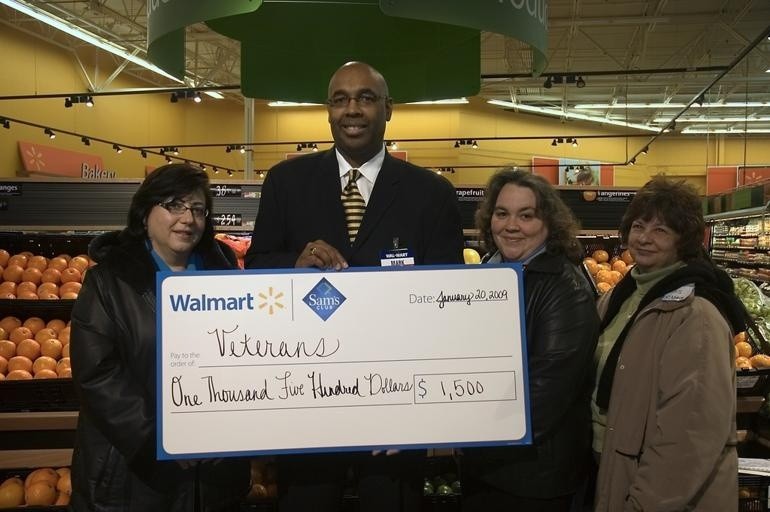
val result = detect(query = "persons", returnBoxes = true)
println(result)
[66,162,254,510]
[591,170,741,512]
[241,59,466,511]
[373,159,603,511]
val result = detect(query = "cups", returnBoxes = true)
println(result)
[327,92,387,109]
[153,199,211,221]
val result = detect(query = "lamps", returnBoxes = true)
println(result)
[141,149,146,157]
[543,76,585,89]
[82,138,89,146]
[187,161,237,173]
[631,159,637,165]
[439,167,454,171]
[114,145,122,152]
[697,96,702,105]
[166,157,171,164]
[225,144,245,153]
[643,148,649,155]
[454,139,478,149]
[2,119,10,128]
[65,95,94,107]
[386,142,396,151]
[566,166,585,173]
[551,138,578,148]
[296,143,318,152]
[46,130,54,138]
[667,123,676,132]
[170,90,201,103]
[160,146,179,154]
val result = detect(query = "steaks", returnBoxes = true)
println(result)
[463,248,480,265]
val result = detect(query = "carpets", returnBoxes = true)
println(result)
[340,168,368,251]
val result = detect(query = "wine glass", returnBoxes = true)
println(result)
[310,246,317,255]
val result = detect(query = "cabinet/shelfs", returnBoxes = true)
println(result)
[0,176,770,512]
